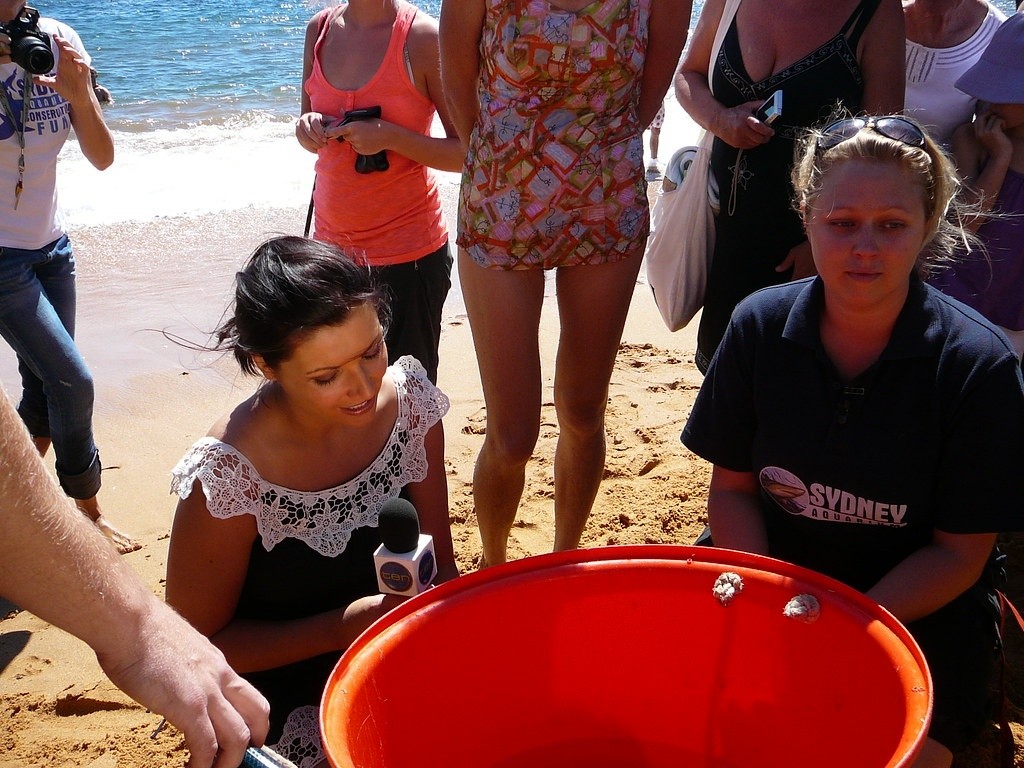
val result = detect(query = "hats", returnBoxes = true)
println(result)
[954,11,1024,104]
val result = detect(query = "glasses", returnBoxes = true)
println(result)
[815,116,928,151]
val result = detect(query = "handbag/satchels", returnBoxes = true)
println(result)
[644,143,715,332]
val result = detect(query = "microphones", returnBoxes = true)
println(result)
[371,499,437,596]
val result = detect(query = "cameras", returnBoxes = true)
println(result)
[755,90,783,126]
[0,5,56,73]
[320,116,351,141]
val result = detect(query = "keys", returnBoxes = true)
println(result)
[14,184,22,210]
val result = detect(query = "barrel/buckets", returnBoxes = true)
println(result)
[318,544,938,768]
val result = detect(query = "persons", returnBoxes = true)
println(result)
[678,112,1024,768]
[162,237,461,767]
[296,0,468,385]
[646,99,663,174]
[438,0,693,569]
[0,0,142,556]
[674,0,1024,557]
[0,384,270,767]
